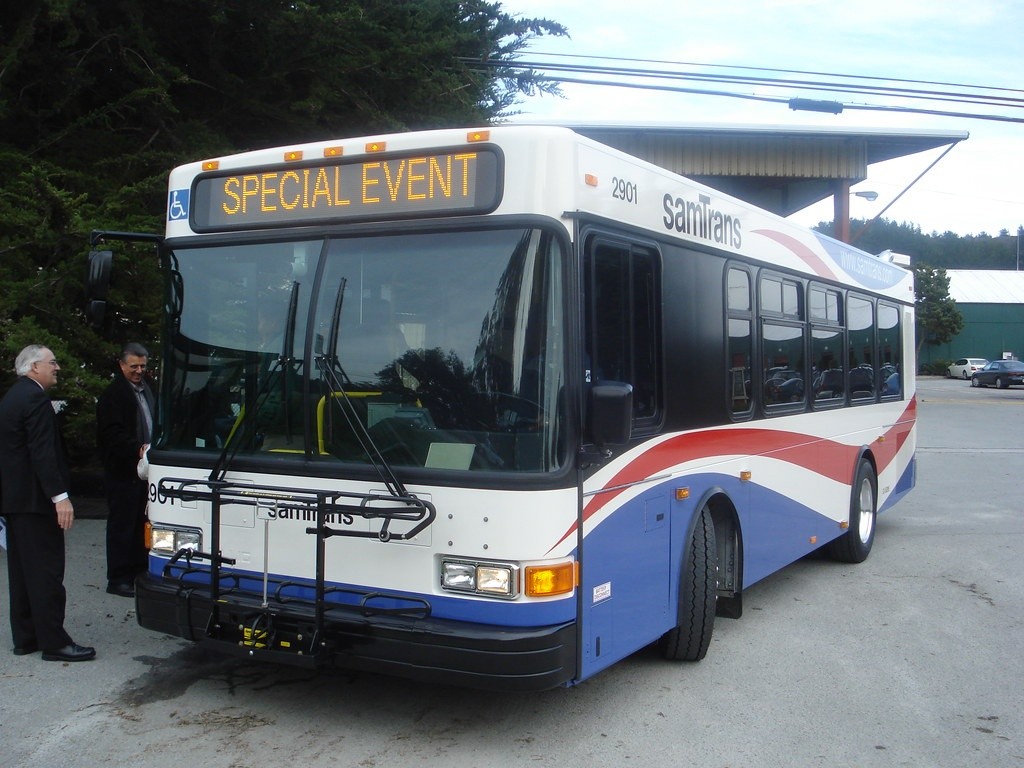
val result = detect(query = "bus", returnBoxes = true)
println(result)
[85,122,918,695]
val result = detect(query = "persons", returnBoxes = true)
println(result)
[1,345,96,662]
[95,341,157,597]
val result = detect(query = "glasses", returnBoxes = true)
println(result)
[35,359,57,367]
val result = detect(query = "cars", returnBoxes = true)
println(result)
[971,359,1024,389]
[745,362,901,406]
[946,357,990,380]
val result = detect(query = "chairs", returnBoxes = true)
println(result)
[744,363,901,420]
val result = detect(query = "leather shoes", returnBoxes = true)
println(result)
[13,637,42,655]
[106,580,135,596]
[41,643,96,662]
